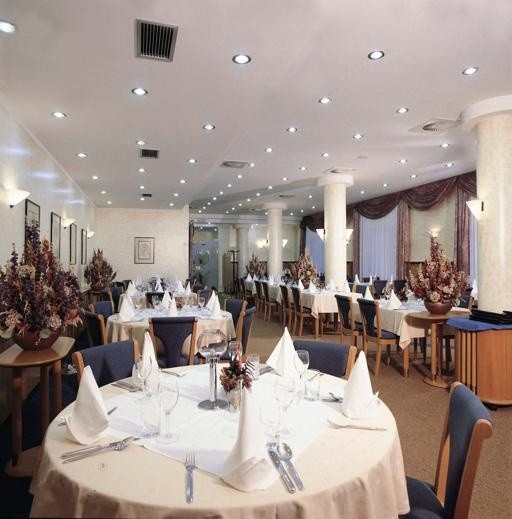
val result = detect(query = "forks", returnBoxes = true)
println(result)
[326,419,388,435]
[184,448,197,504]
[57,405,124,427]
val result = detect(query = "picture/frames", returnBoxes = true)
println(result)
[51,212,61,260]
[70,223,77,265]
[25,198,40,235]
[82,229,87,265]
[134,237,155,264]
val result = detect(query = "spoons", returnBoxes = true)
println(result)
[329,392,345,404]
[59,436,137,465]
[276,442,306,492]
[111,378,142,394]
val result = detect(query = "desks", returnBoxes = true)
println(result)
[0,336,74,477]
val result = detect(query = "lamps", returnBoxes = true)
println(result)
[256,228,354,247]
[6,190,30,207]
[64,219,74,229]
[87,232,95,239]
[466,200,484,221]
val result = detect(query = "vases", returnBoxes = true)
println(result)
[10,323,60,350]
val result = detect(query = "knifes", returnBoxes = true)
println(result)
[268,443,296,493]
[61,435,141,458]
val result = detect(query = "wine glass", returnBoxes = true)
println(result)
[274,377,297,439]
[134,354,153,406]
[121,293,216,322]
[306,281,339,295]
[378,286,422,312]
[129,274,185,292]
[227,340,243,365]
[195,314,232,412]
[293,350,310,396]
[156,381,180,443]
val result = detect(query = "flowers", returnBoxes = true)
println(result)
[84,248,117,287]
[408,234,472,307]
[245,252,267,278]
[0,220,85,346]
[290,246,317,282]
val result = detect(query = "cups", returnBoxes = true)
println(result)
[303,368,321,403]
[245,353,261,381]
